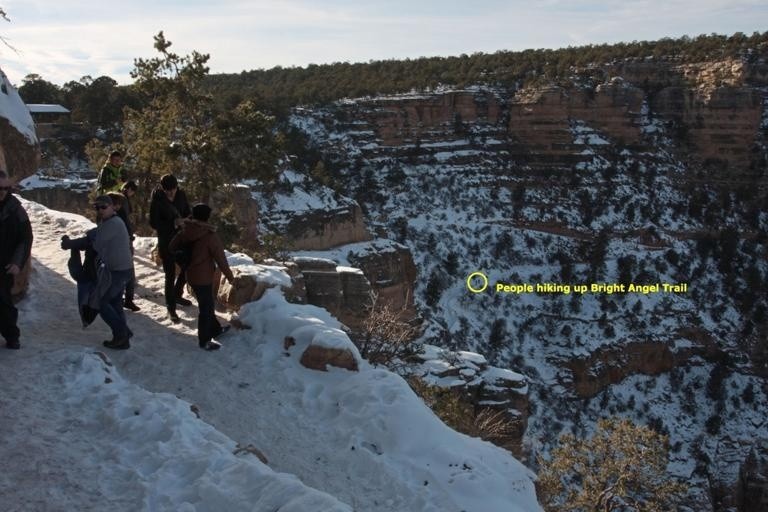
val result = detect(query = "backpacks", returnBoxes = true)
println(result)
[167,232,212,270]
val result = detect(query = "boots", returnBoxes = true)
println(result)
[103,325,133,349]
[175,289,192,305]
[165,290,178,321]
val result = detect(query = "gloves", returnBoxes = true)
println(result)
[61,239,69,250]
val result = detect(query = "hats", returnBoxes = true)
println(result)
[92,195,112,205]
[192,203,211,221]
[106,191,125,204]
[160,173,178,190]
[0,170,12,191]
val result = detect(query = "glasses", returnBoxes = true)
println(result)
[95,206,108,210]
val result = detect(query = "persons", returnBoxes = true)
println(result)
[0,170,31,349]
[167,203,232,349]
[148,174,193,322]
[97,151,121,203]
[59,193,135,350]
[103,181,139,311]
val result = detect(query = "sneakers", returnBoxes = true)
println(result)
[202,341,220,350]
[6,341,20,349]
[124,301,140,311]
[213,325,230,338]
